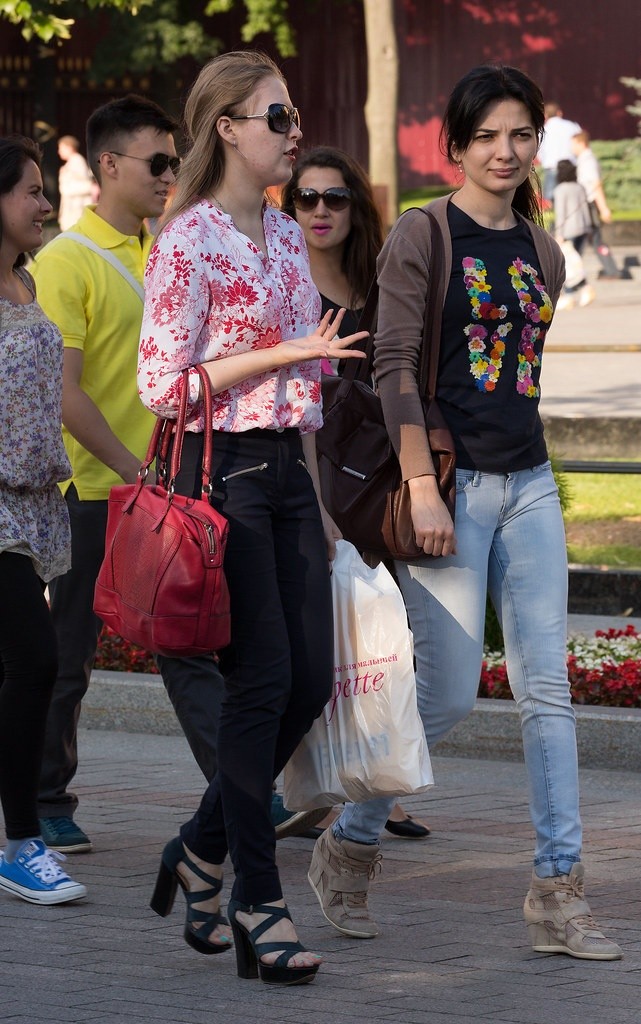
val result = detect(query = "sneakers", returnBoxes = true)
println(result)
[35,814,94,852]
[305,813,384,937]
[0,838,88,905]
[524,861,625,958]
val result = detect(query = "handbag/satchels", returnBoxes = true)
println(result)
[280,540,436,812]
[314,377,455,560]
[558,240,587,288]
[92,365,233,650]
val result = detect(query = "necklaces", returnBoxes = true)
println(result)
[208,190,229,213]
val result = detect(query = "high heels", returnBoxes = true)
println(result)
[228,898,320,986]
[150,833,233,955]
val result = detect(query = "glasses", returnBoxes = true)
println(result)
[229,103,301,133]
[96,152,181,176]
[292,187,352,212]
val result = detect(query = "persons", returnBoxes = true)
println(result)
[570,131,620,279]
[281,144,434,838]
[308,63,623,961]
[57,135,100,232]
[137,52,370,983]
[0,135,87,907]
[536,101,581,202]
[553,160,595,305]
[25,93,328,854]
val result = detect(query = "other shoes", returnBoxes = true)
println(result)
[557,295,571,309]
[385,814,432,837]
[298,826,324,837]
[580,290,596,306]
[270,792,333,840]
